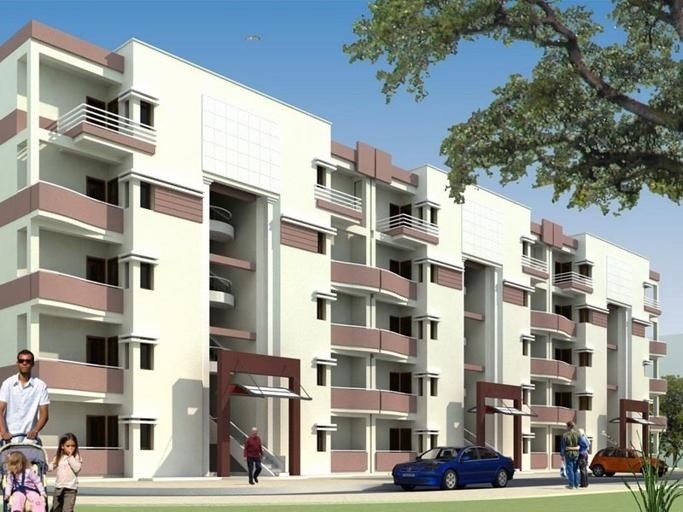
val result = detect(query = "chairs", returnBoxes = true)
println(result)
[442,449,452,461]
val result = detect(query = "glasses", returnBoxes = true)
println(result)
[18,359,33,364]
